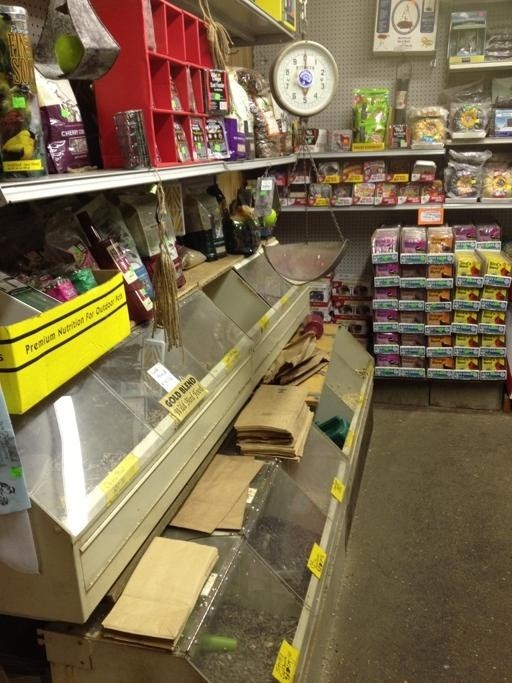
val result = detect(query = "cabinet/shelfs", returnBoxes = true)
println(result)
[93,1,215,166]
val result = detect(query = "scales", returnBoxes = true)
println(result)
[261,0,350,286]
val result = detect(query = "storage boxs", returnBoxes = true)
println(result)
[0,270,132,415]
